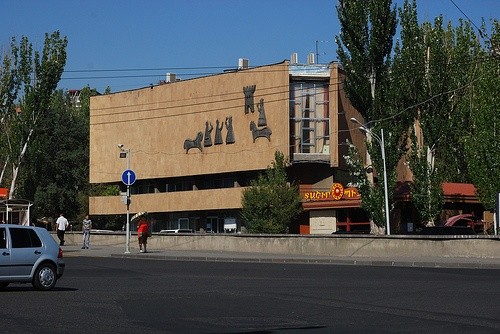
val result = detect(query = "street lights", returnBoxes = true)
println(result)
[350,117,391,235]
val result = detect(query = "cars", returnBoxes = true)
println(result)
[0,224,65,290]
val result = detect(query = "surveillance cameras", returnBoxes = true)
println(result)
[118,143,125,152]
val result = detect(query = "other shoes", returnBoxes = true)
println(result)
[81,246,89,249]
[140,250,146,253]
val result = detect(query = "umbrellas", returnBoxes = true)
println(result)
[130,207,147,221]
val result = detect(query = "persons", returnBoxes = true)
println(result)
[55,213,70,246]
[137,218,148,252]
[81,214,92,250]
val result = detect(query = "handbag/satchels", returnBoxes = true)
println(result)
[147,232,152,237]
